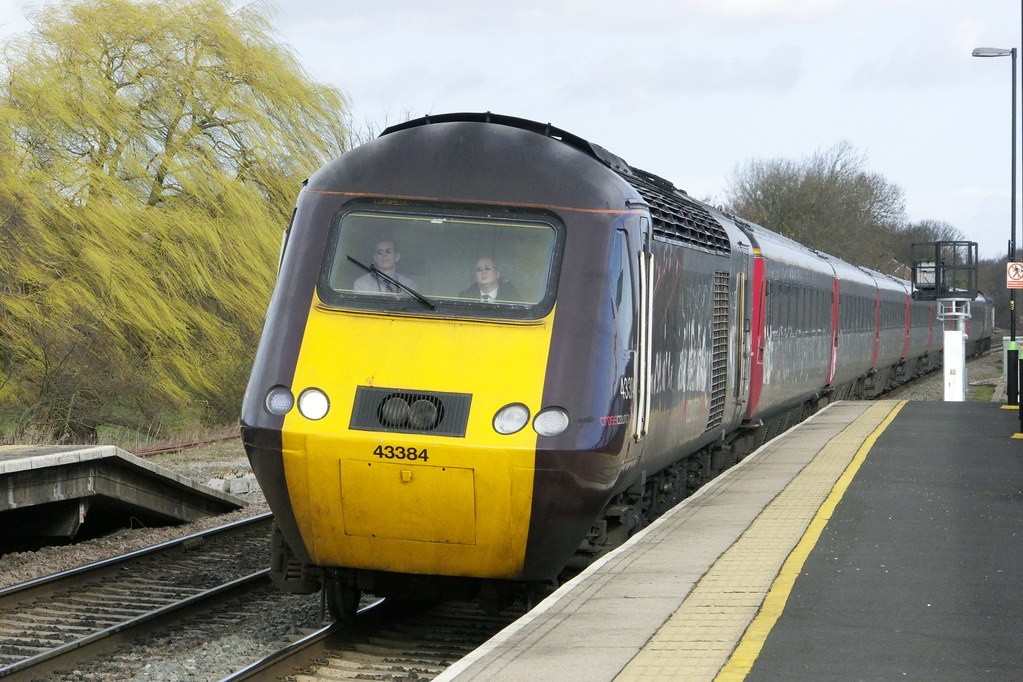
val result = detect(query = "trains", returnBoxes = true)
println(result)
[240,111,996,625]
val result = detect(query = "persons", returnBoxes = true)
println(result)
[458,257,517,304]
[354,237,414,297]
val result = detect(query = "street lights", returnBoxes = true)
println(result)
[972,47,1019,406]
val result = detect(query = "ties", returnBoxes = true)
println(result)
[482,295,489,303]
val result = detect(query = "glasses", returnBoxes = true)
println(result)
[376,248,394,255]
[475,264,494,272]
